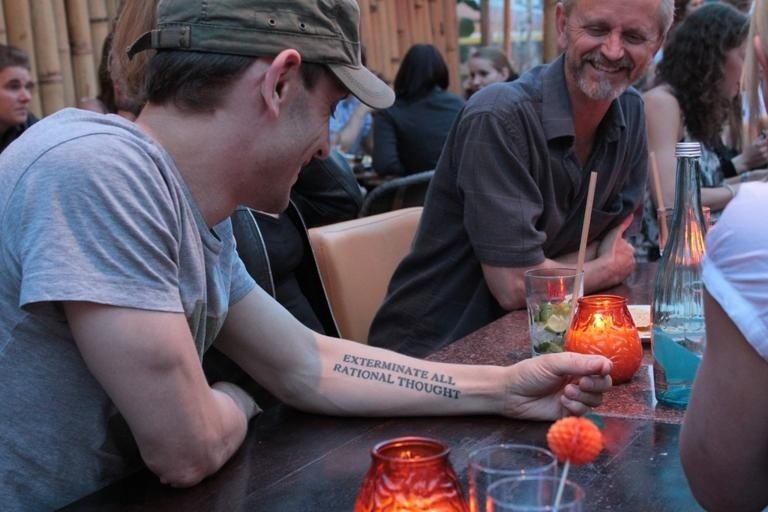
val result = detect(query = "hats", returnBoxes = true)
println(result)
[128,0,402,110]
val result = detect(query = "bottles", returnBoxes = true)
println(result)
[352,435,469,511]
[649,141,712,409]
[566,295,643,386]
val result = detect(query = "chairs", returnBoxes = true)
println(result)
[359,170,435,216]
[231,206,278,301]
[306,203,426,347]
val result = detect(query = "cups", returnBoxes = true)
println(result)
[468,443,585,511]
[523,267,585,355]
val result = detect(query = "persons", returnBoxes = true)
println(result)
[680,2,767,512]
[0,0,614,511]
[109,0,364,377]
[331,43,517,185]
[367,0,677,359]
[0,41,133,148]
[633,0,768,268]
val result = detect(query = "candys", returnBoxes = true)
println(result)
[546,416,604,512]
